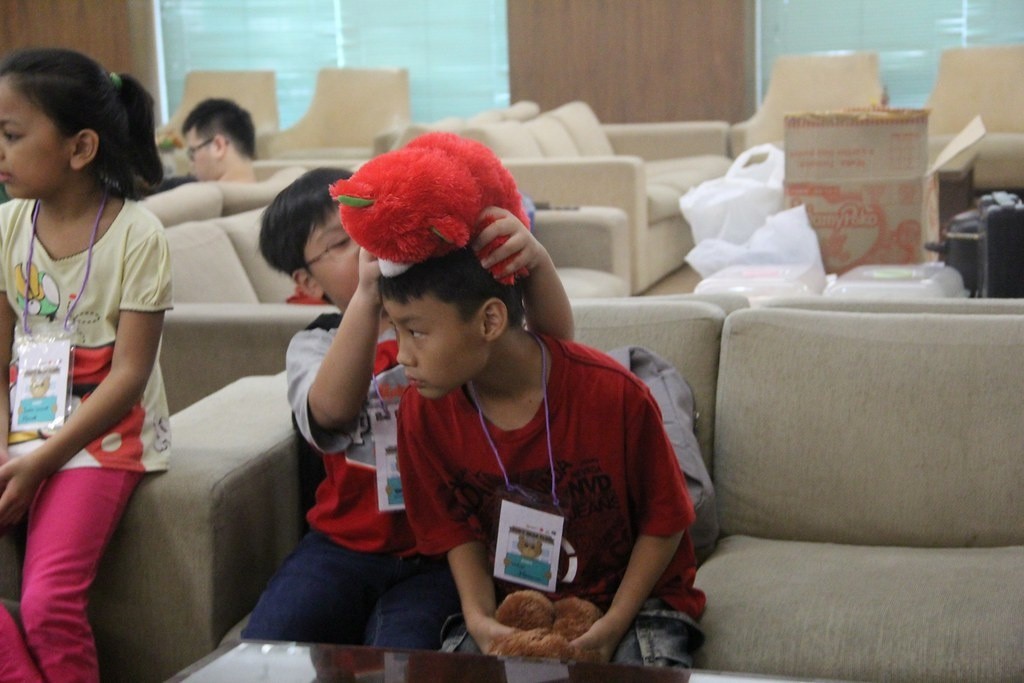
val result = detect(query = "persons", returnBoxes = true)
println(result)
[0,46,173,683]
[239,167,570,649]
[178,96,260,185]
[376,241,709,667]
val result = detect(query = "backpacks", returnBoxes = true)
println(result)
[607,341,722,567]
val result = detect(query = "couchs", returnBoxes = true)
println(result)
[729,50,887,160]
[265,67,414,160]
[0,122,1023,682]
[922,44,1022,189]
[151,68,277,158]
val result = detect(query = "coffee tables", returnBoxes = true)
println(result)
[158,639,856,681]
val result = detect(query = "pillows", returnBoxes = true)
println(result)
[138,101,1022,550]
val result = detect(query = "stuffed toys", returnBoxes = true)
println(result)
[328,131,529,286]
[485,589,607,666]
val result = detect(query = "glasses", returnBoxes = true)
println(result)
[302,234,352,267]
[186,139,229,161]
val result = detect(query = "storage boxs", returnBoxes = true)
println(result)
[781,109,990,280]
